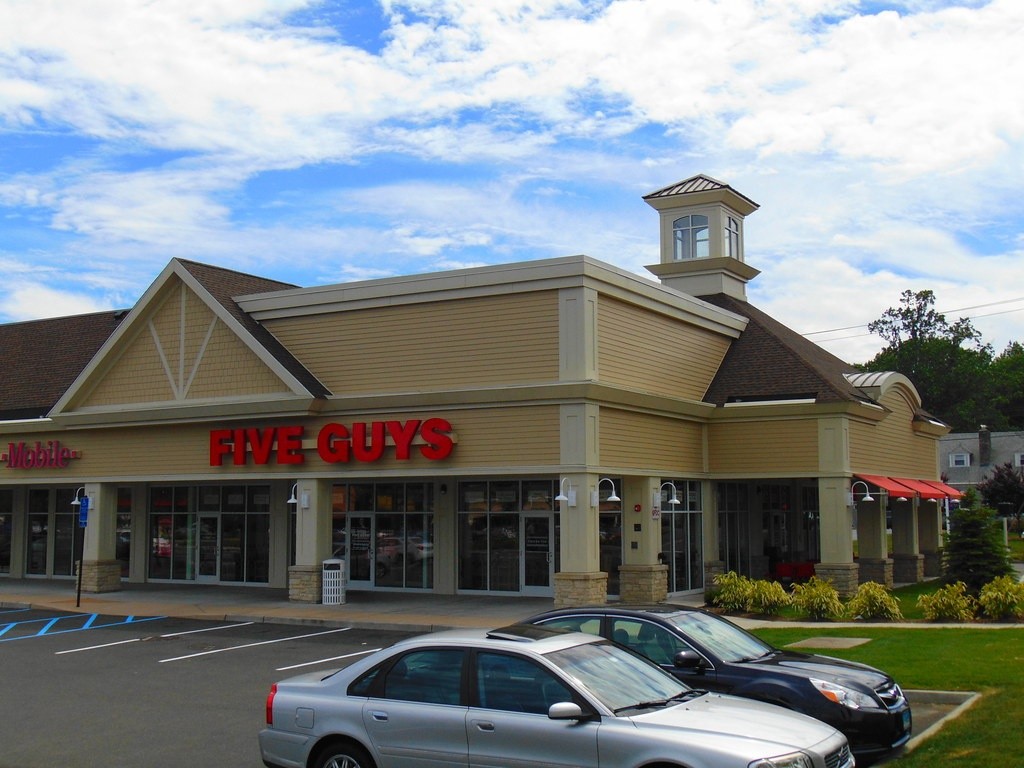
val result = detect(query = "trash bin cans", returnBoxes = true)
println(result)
[322,559,346,606]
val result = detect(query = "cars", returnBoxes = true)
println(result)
[30,522,59,533]
[259,622,857,768]
[116,525,176,560]
[509,602,913,765]
[473,526,512,539]
[331,523,434,577]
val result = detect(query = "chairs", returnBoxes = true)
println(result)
[636,621,674,665]
[484,663,525,713]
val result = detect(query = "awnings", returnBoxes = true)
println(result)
[917,478,966,499]
[854,473,916,498]
[888,476,947,499]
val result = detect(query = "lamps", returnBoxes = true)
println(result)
[949,498,960,505]
[590,477,622,508]
[286,481,311,510]
[846,480,875,508]
[916,495,937,507]
[653,482,681,509]
[885,491,908,506]
[69,487,95,510]
[554,477,577,507]
